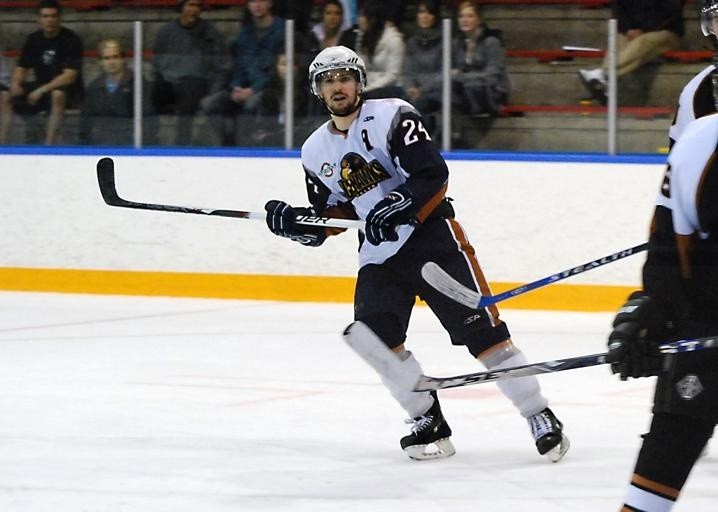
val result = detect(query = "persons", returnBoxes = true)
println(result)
[0,0,84,147]
[669,115,718,292]
[581,0,683,107]
[607,0,718,510]
[78,37,153,147]
[144,0,510,150]
[265,46,570,465]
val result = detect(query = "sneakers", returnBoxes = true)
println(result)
[526,407,562,455]
[399,392,450,449]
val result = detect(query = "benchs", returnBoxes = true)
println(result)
[1,2,715,160]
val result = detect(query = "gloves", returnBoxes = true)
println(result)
[365,183,418,245]
[265,201,324,246]
[607,292,665,381]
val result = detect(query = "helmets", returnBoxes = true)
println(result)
[309,45,367,99]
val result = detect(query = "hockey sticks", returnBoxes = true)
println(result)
[97,158,369,230]
[343,322,718,391]
[422,242,648,310]
